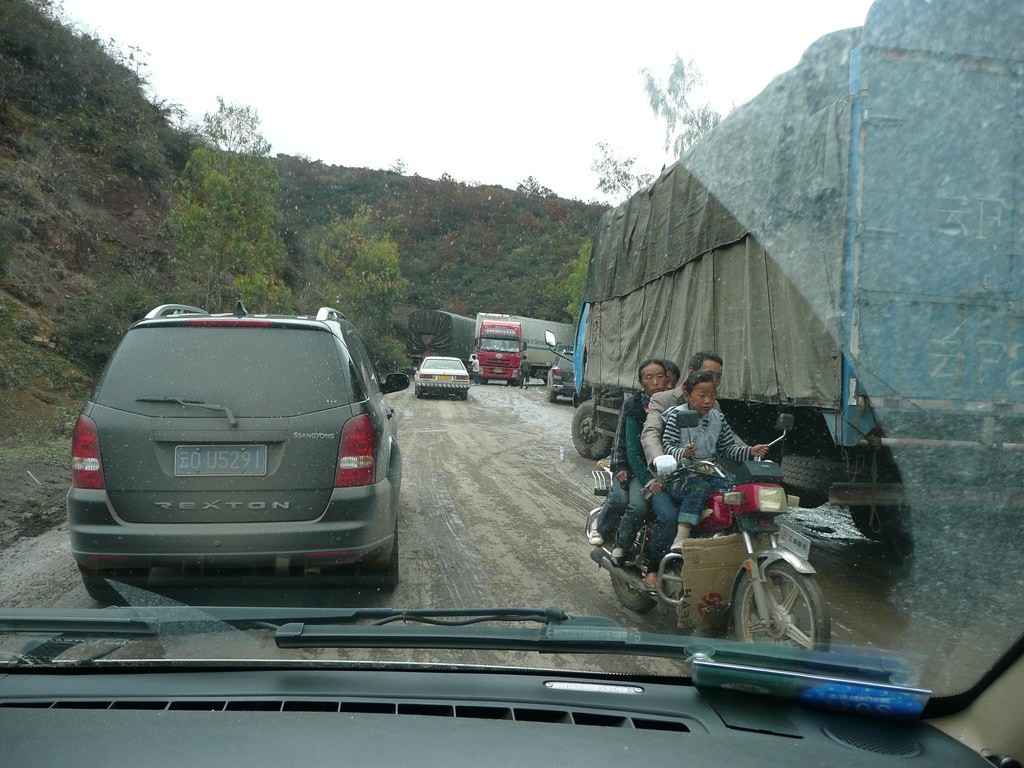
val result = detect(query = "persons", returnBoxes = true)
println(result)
[518,355,531,389]
[471,355,479,386]
[588,351,769,591]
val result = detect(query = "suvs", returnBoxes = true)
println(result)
[67,303,411,600]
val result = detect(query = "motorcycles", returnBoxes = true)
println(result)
[585,412,857,653]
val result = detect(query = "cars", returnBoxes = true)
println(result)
[545,348,576,403]
[412,355,471,401]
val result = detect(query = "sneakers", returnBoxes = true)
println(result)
[589,518,604,545]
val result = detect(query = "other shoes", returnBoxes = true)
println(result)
[669,544,683,555]
[645,571,664,591]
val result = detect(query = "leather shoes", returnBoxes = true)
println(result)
[610,543,625,568]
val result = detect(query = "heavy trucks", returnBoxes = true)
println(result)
[470,313,575,386]
[544,45,1024,547]
